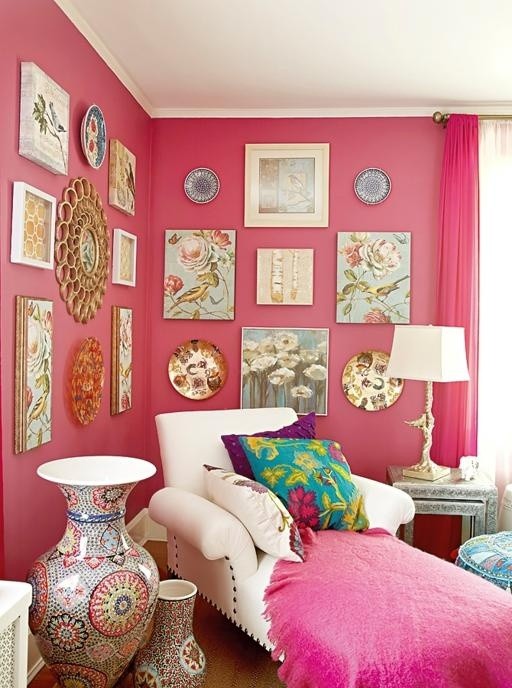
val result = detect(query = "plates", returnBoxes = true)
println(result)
[71,337,106,426]
[168,340,229,400]
[81,103,107,171]
[184,168,221,205]
[354,168,390,204]
[340,350,405,412]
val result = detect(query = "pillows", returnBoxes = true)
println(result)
[239,433,372,533]
[199,463,303,568]
[213,410,318,482]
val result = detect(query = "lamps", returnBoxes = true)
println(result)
[384,324,475,483]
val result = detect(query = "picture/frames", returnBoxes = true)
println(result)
[8,180,58,275]
[238,324,332,420]
[242,138,331,230]
[109,224,141,292]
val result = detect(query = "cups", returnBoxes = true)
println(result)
[460,456,479,480]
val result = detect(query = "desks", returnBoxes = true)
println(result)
[386,461,501,565]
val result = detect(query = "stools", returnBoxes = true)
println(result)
[450,529,511,587]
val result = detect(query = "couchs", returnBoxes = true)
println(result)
[142,394,512,687]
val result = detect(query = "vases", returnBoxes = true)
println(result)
[23,451,169,687]
[126,558,212,688]
